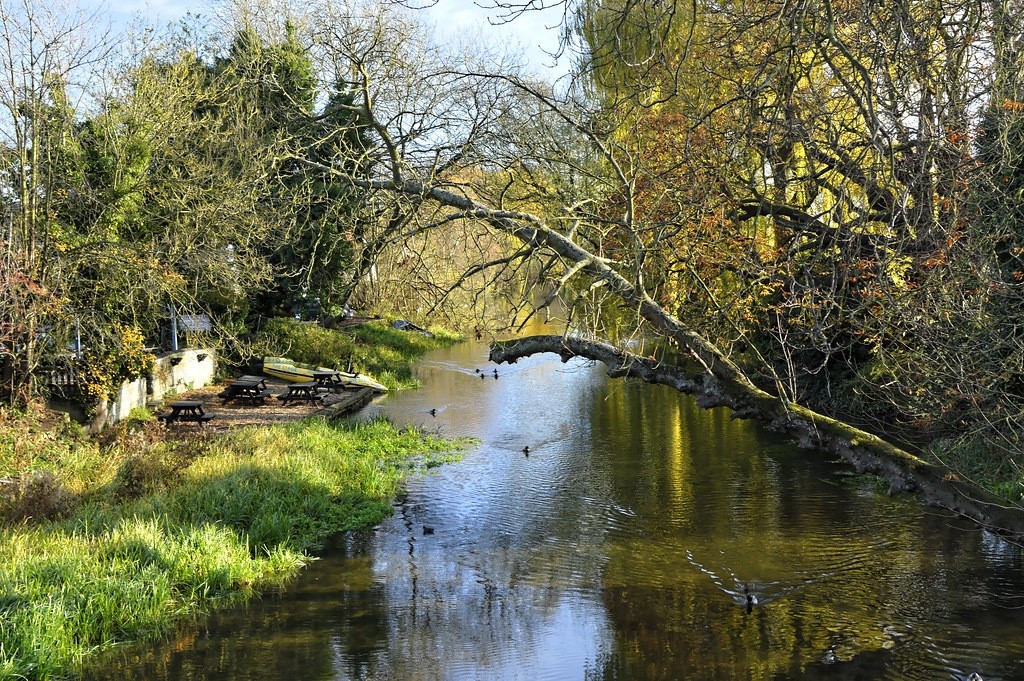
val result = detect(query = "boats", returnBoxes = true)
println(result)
[262,356,388,391]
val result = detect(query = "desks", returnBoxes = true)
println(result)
[222,381,265,407]
[313,372,347,394]
[229,375,272,398]
[282,382,325,407]
[166,400,204,429]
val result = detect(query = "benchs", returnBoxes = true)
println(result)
[276,392,291,401]
[158,411,172,422]
[336,381,351,386]
[217,387,232,398]
[257,388,276,399]
[201,413,217,423]
[313,392,330,400]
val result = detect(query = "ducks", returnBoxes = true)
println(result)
[430,408,436,413]
[422,525,435,534]
[476,369,484,378]
[523,446,531,452]
[493,369,498,377]
[748,594,758,606]
